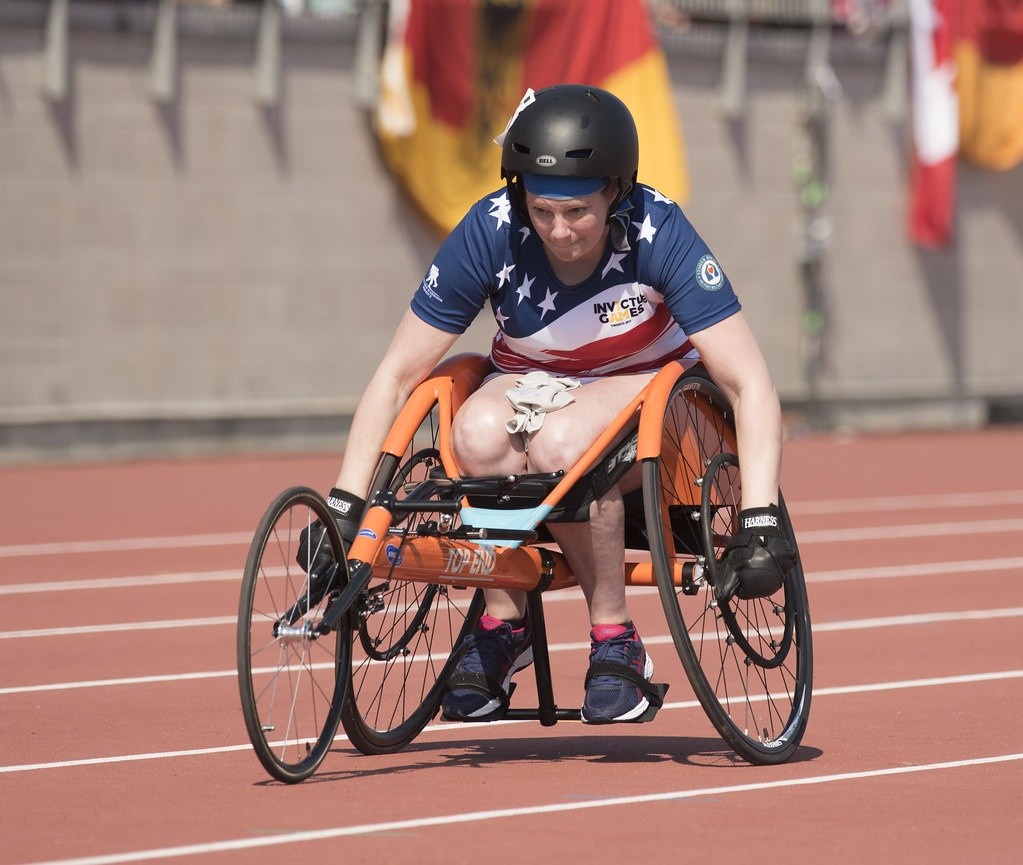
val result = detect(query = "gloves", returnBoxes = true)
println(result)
[295,488,366,577]
[715,503,798,600]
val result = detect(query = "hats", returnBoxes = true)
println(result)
[521,149,610,201]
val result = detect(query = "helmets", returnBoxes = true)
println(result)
[503,84,641,206]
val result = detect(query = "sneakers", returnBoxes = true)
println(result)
[441,614,533,718]
[580,624,655,725]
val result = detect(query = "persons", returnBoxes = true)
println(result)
[296,82,796,727]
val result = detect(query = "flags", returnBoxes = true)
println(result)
[910,1,1023,250]
[370,0,684,238]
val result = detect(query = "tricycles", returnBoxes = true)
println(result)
[233,349,817,785]
